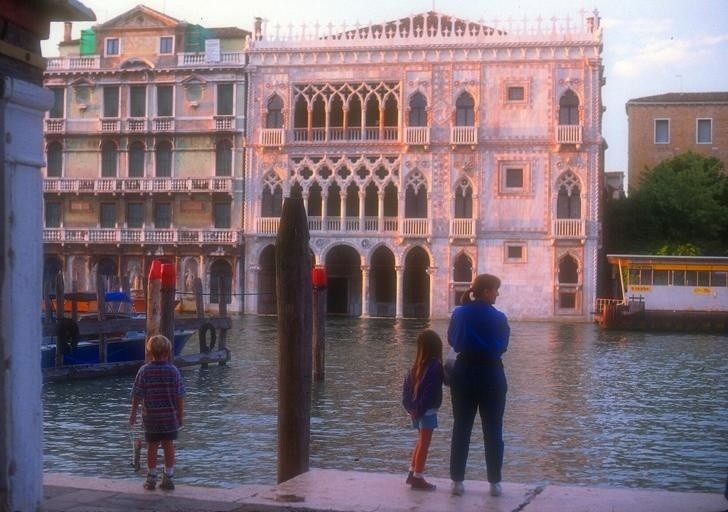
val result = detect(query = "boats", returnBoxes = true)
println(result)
[606,254,727,332]
[40,290,195,367]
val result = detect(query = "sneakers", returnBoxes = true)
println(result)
[143,471,156,491]
[450,480,466,497]
[488,482,503,498]
[404,470,437,490]
[158,472,176,492]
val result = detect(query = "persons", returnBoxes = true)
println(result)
[185,267,194,291]
[129,334,188,494]
[400,330,444,491]
[447,273,511,497]
